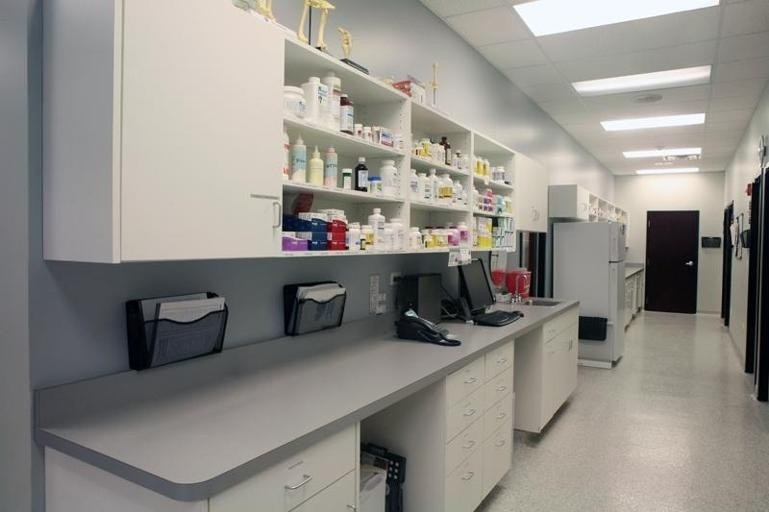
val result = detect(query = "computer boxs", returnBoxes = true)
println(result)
[398,273,441,323]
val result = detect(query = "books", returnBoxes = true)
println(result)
[287,283,349,336]
[136,288,227,369]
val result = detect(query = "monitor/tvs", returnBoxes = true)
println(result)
[457,257,494,316]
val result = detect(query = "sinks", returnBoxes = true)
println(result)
[523,299,561,306]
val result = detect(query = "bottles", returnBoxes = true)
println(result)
[354,122,372,141]
[410,136,470,250]
[369,160,398,197]
[282,125,338,188]
[475,155,505,184]
[356,156,369,191]
[360,205,404,252]
[340,95,354,135]
[342,168,351,189]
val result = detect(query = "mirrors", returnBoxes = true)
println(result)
[489,250,509,284]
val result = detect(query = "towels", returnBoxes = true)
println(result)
[578,315,609,341]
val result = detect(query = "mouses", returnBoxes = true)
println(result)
[513,311,524,317]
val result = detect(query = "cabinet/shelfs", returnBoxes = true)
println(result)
[43,421,360,512]
[369,340,517,512]
[548,185,630,247]
[39,0,287,265]
[623,270,644,329]
[282,38,518,255]
[513,303,580,433]
[516,152,550,234]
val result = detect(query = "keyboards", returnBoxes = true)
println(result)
[474,310,519,326]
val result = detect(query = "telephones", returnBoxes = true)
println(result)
[394,308,449,343]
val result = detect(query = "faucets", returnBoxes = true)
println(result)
[515,273,528,300]
